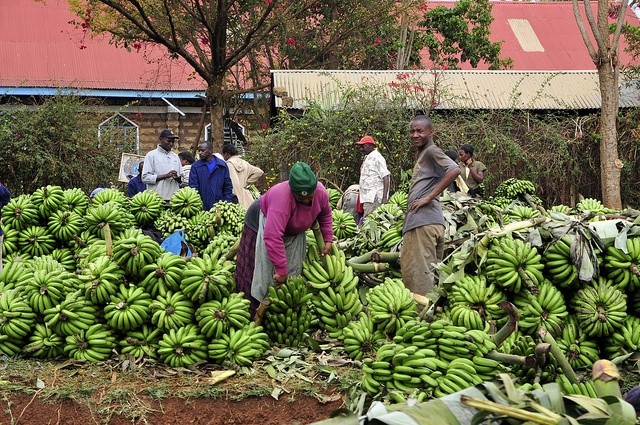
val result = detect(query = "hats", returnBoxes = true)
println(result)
[289,161,317,196]
[355,135,375,145]
[160,129,179,139]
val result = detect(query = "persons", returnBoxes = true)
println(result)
[399,114,462,297]
[127,160,146,198]
[222,144,264,212]
[177,150,197,187]
[1,182,11,208]
[141,130,185,211]
[89,180,109,199]
[354,136,391,218]
[336,184,360,225]
[232,162,333,323]
[458,143,488,198]
[188,140,234,211]
[444,150,460,193]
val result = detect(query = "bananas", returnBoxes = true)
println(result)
[266,242,364,348]
[1,184,269,369]
[326,188,341,210]
[343,177,640,405]
[331,210,357,239]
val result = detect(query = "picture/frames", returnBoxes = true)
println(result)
[117,152,145,183]
